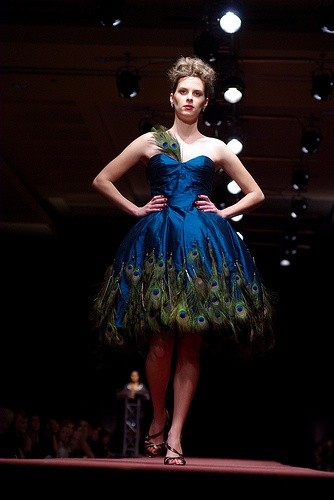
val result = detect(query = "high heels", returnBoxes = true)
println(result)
[164,442,186,465]
[143,410,169,457]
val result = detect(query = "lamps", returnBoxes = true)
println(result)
[310,68,334,100]
[279,232,297,267]
[221,172,242,195]
[299,123,324,154]
[222,74,246,103]
[227,133,245,155]
[203,101,224,127]
[218,0,245,35]
[193,34,218,63]
[319,0,334,36]
[288,199,310,219]
[116,64,140,100]
[291,164,313,192]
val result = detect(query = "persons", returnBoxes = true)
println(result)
[115,369,151,400]
[305,425,334,470]
[91,56,265,464]
[0,410,114,459]
